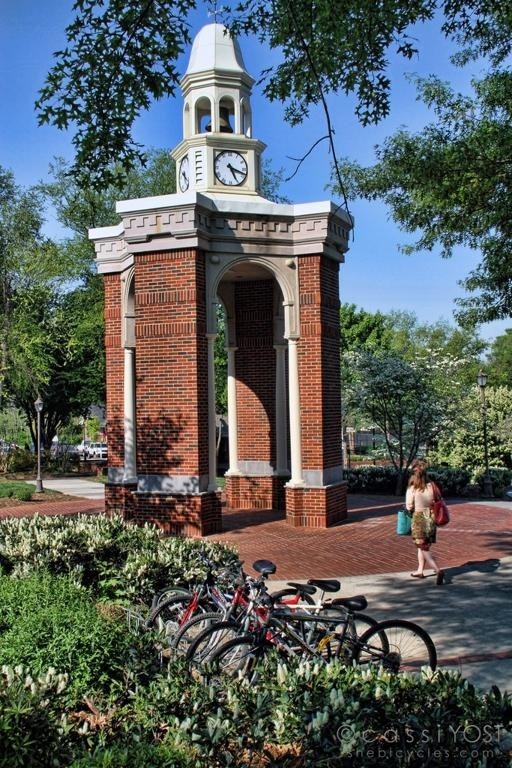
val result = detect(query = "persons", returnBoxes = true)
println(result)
[405,466,444,586]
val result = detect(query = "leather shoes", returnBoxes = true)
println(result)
[412,573,423,577]
[437,571,445,585]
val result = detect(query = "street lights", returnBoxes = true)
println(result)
[477,369,495,499]
[82,423,85,461]
[34,396,44,492]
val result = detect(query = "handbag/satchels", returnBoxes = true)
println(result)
[396,509,412,536]
[432,499,449,527]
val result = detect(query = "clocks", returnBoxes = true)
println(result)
[212,149,249,188]
[178,157,192,192]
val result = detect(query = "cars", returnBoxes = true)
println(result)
[30,441,108,460]
